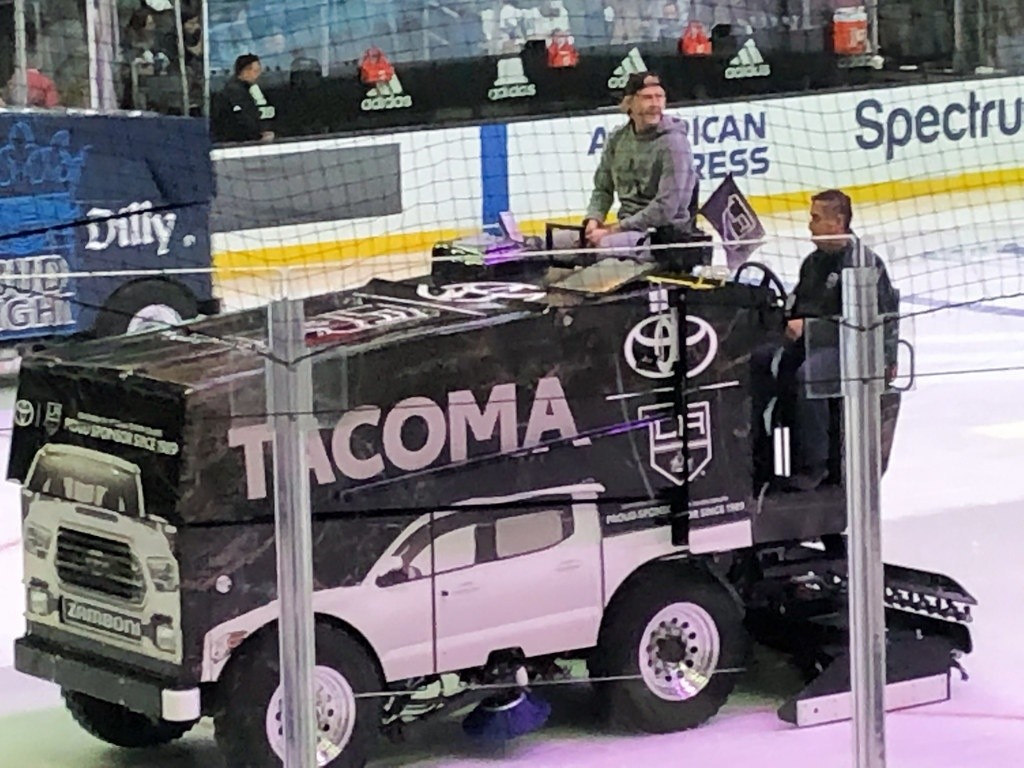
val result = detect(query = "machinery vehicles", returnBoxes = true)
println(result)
[10,218,979,768]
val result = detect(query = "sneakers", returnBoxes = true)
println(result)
[784,468,830,492]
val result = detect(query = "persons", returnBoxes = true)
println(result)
[582,73,699,269]
[119,1,200,116]
[4,28,59,106]
[749,188,901,489]
[212,53,277,143]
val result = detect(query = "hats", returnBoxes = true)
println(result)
[624,71,666,93]
